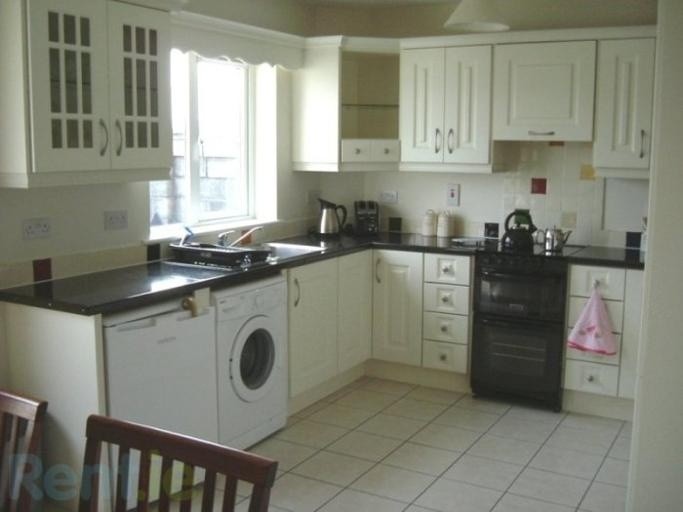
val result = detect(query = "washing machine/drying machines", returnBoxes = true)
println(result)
[209,274,289,451]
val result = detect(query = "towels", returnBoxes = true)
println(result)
[568,289,617,355]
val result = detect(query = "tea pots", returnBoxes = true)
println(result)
[500,210,536,250]
[537,225,571,251]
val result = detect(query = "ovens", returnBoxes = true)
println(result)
[470,269,569,412]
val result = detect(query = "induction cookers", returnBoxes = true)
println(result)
[476,239,585,276]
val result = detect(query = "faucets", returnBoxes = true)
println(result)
[218,225,264,247]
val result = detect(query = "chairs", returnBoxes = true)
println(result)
[78,414,279,512]
[0,389,48,512]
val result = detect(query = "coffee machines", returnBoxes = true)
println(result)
[354,200,378,238]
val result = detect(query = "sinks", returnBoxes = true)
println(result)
[252,241,328,262]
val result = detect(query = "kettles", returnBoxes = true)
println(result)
[314,198,347,239]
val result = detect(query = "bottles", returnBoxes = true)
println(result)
[437,209,453,236]
[421,210,436,238]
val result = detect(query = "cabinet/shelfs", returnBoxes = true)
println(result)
[492,28,597,142]
[0,0,174,189]
[337,249,372,390]
[562,265,643,423]
[593,26,656,179]
[372,247,474,392]
[287,257,337,416]
[399,34,508,172]
[292,36,399,172]
